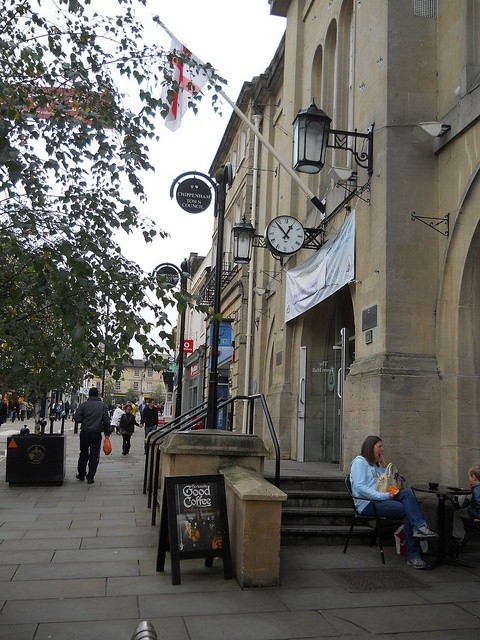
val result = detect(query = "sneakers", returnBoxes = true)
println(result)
[413,524,439,537]
[406,556,427,569]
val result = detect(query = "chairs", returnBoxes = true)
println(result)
[345,473,399,564]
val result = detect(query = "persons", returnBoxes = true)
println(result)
[73,388,110,483]
[130,391,146,416]
[156,401,166,414]
[48,399,71,421]
[140,399,158,454]
[350,436,441,569]
[109,403,124,435]
[0,395,35,423]
[451,464,480,547]
[119,405,141,456]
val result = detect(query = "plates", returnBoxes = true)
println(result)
[447,486,469,491]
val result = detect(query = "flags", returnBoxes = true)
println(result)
[160,37,210,131]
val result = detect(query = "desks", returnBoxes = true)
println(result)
[410,484,472,569]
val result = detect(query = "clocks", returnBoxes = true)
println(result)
[264,215,305,255]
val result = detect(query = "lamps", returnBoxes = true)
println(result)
[408,122,451,144]
[253,288,276,300]
[328,166,357,185]
[231,213,283,265]
[292,97,375,176]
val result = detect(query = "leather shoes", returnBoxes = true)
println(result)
[88,479,93,483]
[76,472,84,480]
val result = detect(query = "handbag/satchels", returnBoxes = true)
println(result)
[394,524,428,555]
[378,463,399,492]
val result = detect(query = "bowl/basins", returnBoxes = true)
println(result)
[428,481,438,487]
